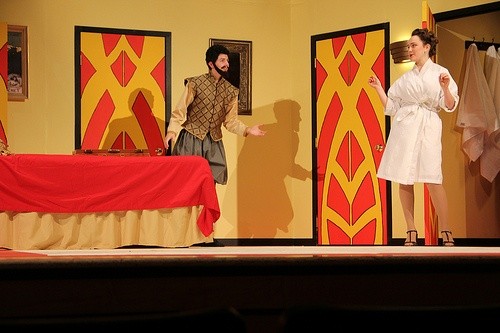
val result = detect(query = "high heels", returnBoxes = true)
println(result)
[441,230,455,246]
[404,230,419,246]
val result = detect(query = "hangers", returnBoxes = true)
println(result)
[465,39,500,53]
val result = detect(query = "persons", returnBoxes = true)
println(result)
[366,27,460,246]
[163,44,267,189]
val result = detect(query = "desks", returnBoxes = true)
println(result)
[1,152,220,253]
